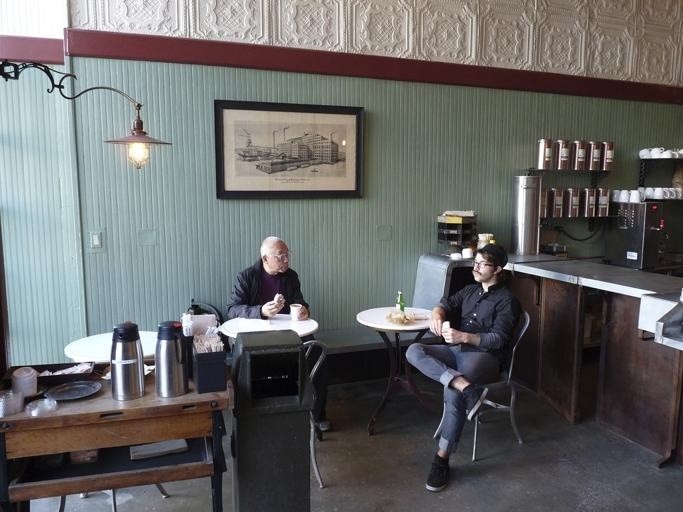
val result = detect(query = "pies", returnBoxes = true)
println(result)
[275,293,285,304]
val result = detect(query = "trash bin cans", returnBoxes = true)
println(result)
[231,330,315,512]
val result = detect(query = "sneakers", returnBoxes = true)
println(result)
[425,458,450,492]
[466,384,488,421]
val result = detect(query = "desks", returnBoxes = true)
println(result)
[354,306,441,439]
[0,356,231,512]
[65,330,180,512]
[219,313,334,491]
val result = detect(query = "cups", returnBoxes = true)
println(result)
[12,366,38,397]
[289,303,302,322]
[539,186,610,217]
[536,137,616,172]
[638,146,682,159]
[450,248,473,260]
[637,185,683,200]
[0,389,23,418]
[611,189,641,204]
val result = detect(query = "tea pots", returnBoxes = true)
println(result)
[108,320,146,403]
[153,320,191,398]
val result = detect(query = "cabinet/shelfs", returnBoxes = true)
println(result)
[529,168,622,221]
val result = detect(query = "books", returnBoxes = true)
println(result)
[127,439,189,461]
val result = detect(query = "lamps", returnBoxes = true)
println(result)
[1,57,174,169]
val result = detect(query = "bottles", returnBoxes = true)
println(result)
[395,290,405,311]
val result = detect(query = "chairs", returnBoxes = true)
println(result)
[303,339,328,491]
[434,309,530,461]
[413,251,453,309]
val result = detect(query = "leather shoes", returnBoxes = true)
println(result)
[311,414,332,432]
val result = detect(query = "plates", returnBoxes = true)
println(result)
[43,380,102,401]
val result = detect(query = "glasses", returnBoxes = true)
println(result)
[265,253,289,259]
[472,260,496,268]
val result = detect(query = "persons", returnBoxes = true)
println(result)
[226,234,336,434]
[403,243,523,494]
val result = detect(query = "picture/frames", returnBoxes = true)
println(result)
[214,98,365,199]
